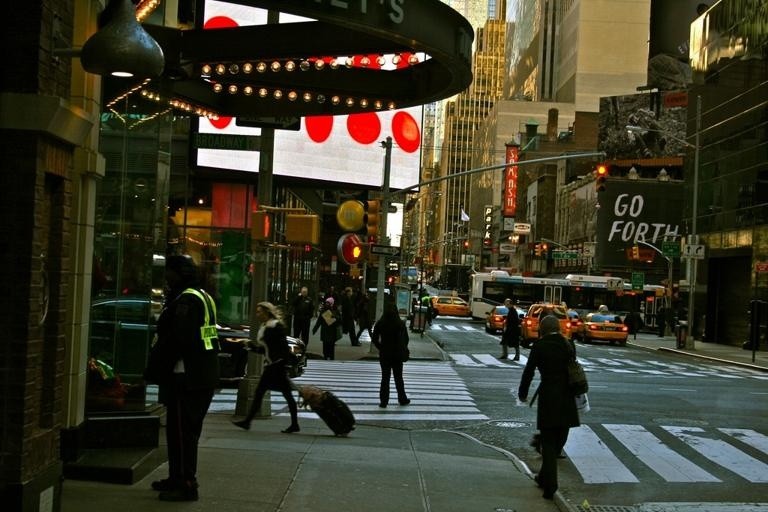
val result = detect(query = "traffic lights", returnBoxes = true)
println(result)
[632,246,639,260]
[363,199,381,235]
[535,243,541,257]
[251,210,275,244]
[542,243,548,255]
[596,164,608,192]
[464,242,468,253]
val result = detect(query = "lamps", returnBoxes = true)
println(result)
[80,0,166,78]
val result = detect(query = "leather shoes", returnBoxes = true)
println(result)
[281,423,301,434]
[497,354,520,360]
[231,421,252,429]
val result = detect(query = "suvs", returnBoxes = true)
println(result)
[430,295,471,318]
[518,303,573,348]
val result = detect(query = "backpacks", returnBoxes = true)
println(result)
[309,392,357,437]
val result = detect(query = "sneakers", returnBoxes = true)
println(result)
[399,398,410,406]
[534,475,558,501]
[379,400,390,408]
[152,476,200,502]
[352,340,363,347]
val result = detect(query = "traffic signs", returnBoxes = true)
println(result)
[194,132,261,151]
[371,244,403,256]
[552,253,577,259]
[235,116,301,131]
[662,241,681,257]
[631,272,643,291]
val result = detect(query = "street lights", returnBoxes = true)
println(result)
[626,94,704,352]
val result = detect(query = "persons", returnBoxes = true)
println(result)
[232,301,300,432]
[291,286,314,346]
[519,316,580,499]
[152,254,220,502]
[500,298,519,361]
[421,293,433,326]
[313,286,376,360]
[372,304,410,407]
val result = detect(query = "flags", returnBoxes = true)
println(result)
[461,209,470,221]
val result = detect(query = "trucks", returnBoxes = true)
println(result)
[400,266,418,290]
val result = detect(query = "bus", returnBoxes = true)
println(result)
[469,269,669,336]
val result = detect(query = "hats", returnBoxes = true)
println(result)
[325,296,336,308]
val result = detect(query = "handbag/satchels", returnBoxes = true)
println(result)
[393,321,410,364]
[333,323,344,341]
[567,359,588,395]
[298,383,321,410]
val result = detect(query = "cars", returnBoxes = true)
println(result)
[485,303,524,334]
[566,308,584,341]
[577,305,630,346]
[89,298,308,389]
